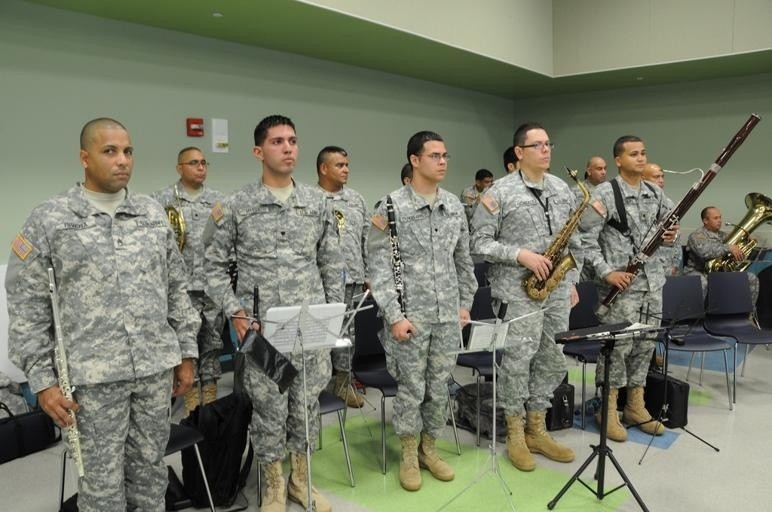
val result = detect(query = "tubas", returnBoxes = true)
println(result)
[710,194,772,274]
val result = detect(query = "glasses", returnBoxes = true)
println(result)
[520,142,556,150]
[179,161,207,165]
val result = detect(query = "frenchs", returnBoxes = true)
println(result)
[163,186,186,252]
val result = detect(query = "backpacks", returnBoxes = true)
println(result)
[453,378,509,444]
[181,350,256,510]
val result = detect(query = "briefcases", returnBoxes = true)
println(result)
[548,384,574,430]
[643,370,690,429]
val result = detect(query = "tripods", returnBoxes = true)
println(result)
[626,303,720,465]
[547,338,650,512]
[340,309,376,441]
[434,308,516,512]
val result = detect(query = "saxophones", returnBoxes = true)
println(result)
[523,169,591,298]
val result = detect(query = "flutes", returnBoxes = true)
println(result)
[47,268,83,475]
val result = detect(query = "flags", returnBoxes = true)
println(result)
[11,234,32,260]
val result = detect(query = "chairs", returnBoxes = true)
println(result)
[350,299,462,475]
[685,272,770,382]
[563,281,609,431]
[216,309,356,509]
[457,284,501,446]
[56,428,214,512]
[661,275,733,412]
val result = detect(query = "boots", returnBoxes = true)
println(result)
[622,386,665,436]
[286,450,331,512]
[504,413,537,471]
[201,382,218,404]
[417,431,455,481]
[399,436,423,491]
[335,367,365,409]
[184,385,200,412]
[595,386,628,442]
[259,460,287,512]
[526,410,576,463]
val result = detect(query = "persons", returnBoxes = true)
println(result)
[310,144,369,408]
[204,117,346,512]
[461,121,682,472]
[686,206,760,328]
[154,147,237,412]
[364,131,478,490]
[4,117,202,512]
